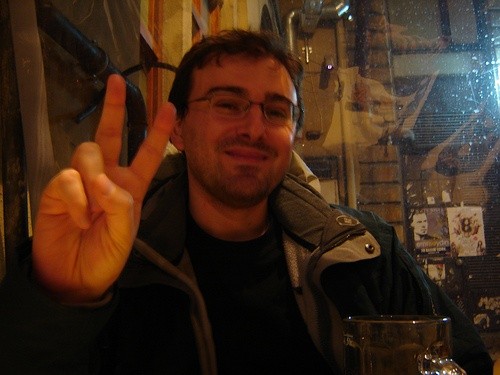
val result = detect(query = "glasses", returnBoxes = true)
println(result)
[187,91,301,126]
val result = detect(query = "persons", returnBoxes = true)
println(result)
[2,29,494,373]
[410,209,438,240]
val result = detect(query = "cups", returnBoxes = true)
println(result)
[343,315,467,375]
